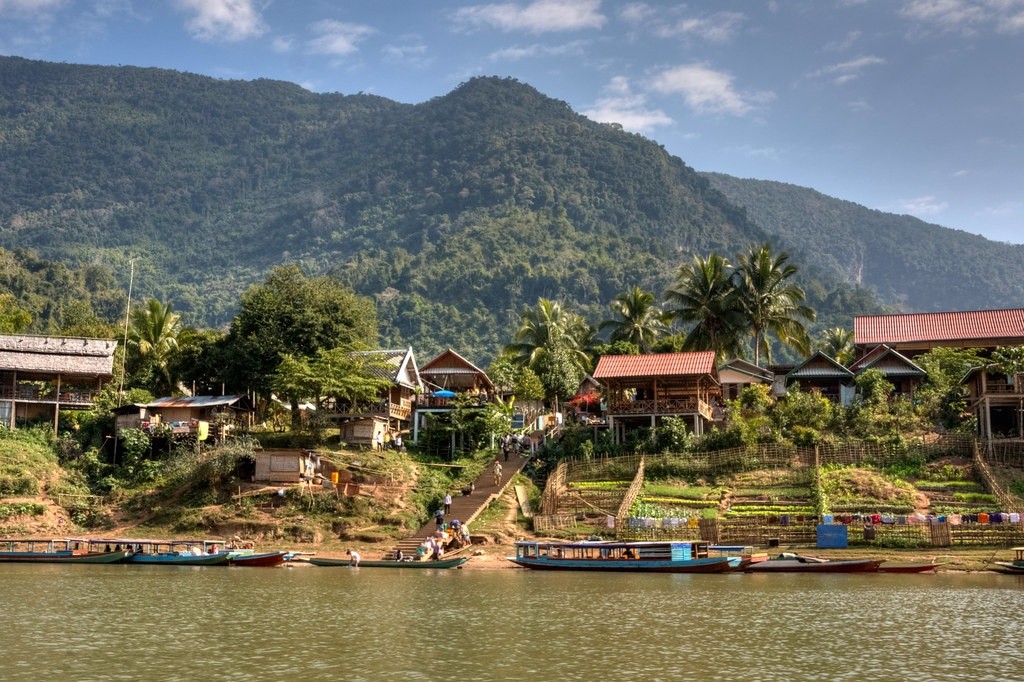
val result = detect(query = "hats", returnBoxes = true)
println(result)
[494,460,500,464]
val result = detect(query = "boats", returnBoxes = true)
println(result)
[736,552,889,572]
[695,544,770,567]
[995,546,1024,571]
[503,540,743,572]
[308,556,472,567]
[878,560,947,573]
[0,536,139,565]
[986,567,1024,574]
[84,536,316,567]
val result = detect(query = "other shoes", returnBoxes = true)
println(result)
[445,515,447,516]
[448,514,450,515]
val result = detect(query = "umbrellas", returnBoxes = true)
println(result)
[433,390,456,397]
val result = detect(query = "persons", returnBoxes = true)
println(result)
[394,549,403,562]
[480,388,487,398]
[460,522,469,545]
[137,545,143,553]
[347,550,360,567]
[503,443,509,461]
[104,545,110,552]
[444,491,452,516]
[468,482,475,495]
[435,508,443,532]
[492,460,502,487]
[384,432,402,451]
[498,431,531,453]
[713,396,726,407]
[127,545,133,552]
[115,545,120,550]
[377,431,383,452]
[623,548,635,558]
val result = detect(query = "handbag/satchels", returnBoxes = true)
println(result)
[512,438,517,443]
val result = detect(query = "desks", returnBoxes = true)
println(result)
[698,545,753,557]
[1010,547,1024,560]
[157,552,179,556]
